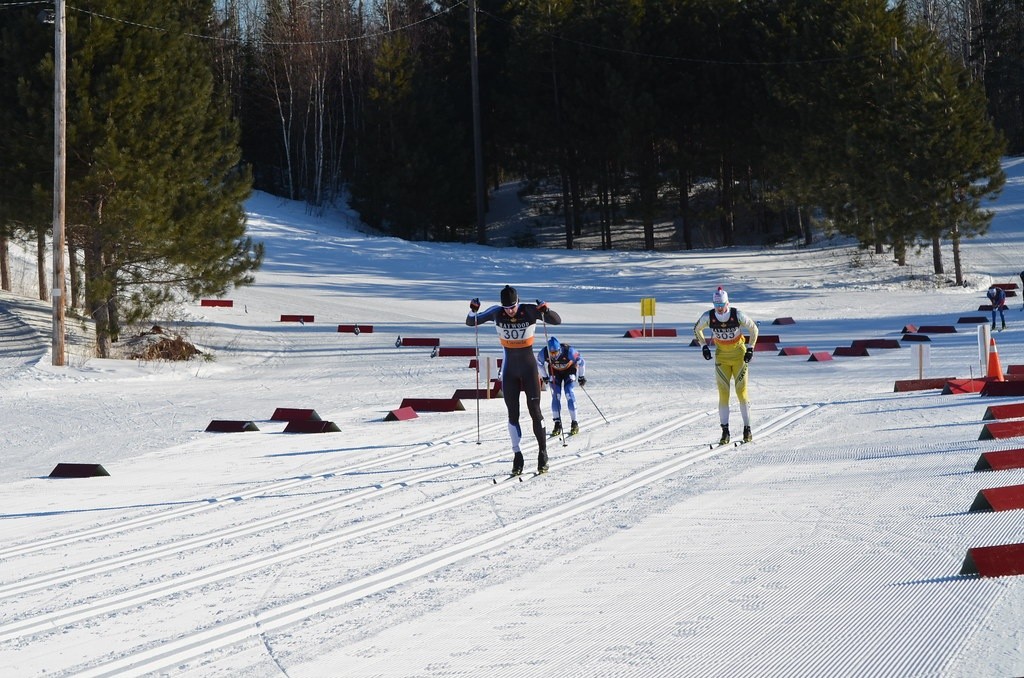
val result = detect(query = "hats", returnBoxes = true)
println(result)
[548,336,561,352]
[988,286,996,294]
[500,285,518,307]
[713,286,729,304]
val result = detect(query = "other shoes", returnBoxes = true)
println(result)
[1002,322,1006,330]
[992,322,996,330]
[743,426,752,442]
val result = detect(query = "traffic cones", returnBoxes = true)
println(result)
[985,338,1008,382]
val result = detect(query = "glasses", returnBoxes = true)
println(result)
[713,303,725,307]
[503,302,518,309]
[549,351,559,355]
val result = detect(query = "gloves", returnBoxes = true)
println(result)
[536,299,547,312]
[702,344,713,360]
[470,299,481,313]
[578,375,587,386]
[542,375,550,384]
[744,346,754,363]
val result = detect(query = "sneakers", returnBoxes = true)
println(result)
[719,423,730,445]
[538,449,549,471]
[552,422,562,436]
[512,451,524,474]
[570,420,579,435]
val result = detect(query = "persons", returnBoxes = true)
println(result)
[694,287,758,445]
[1020,271,1024,311]
[536,337,587,436]
[987,286,1006,332]
[466,284,561,477]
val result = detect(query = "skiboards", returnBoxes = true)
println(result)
[710,441,746,450]
[990,328,1005,332]
[1020,308,1024,311]
[493,473,543,484]
[547,435,574,441]
